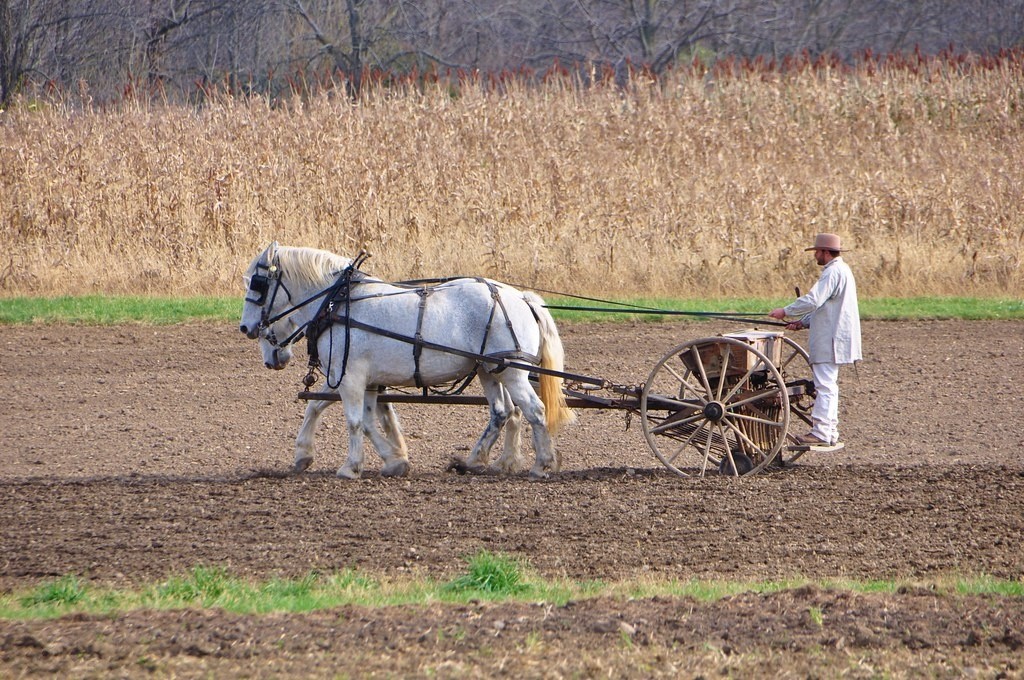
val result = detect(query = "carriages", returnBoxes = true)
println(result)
[237,240,845,481]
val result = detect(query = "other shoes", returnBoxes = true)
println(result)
[795,433,830,447]
[830,441,836,446]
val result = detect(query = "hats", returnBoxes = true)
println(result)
[804,233,850,252]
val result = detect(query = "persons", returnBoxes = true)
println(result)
[767,233,864,448]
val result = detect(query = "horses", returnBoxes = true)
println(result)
[238,240,577,483]
